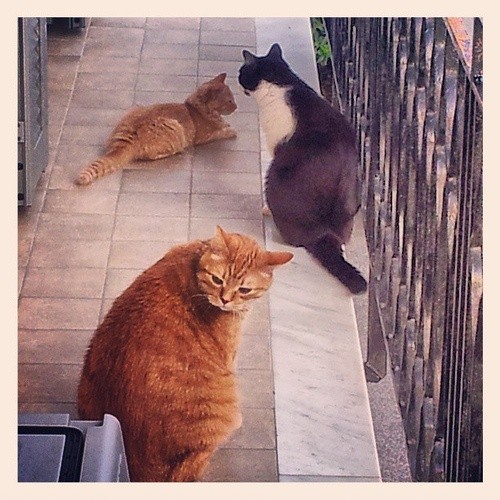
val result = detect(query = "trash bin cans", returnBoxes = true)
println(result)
[18,413,130,483]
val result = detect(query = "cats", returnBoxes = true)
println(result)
[74,226,294,482]
[74,72,238,186]
[236,42,367,293]
